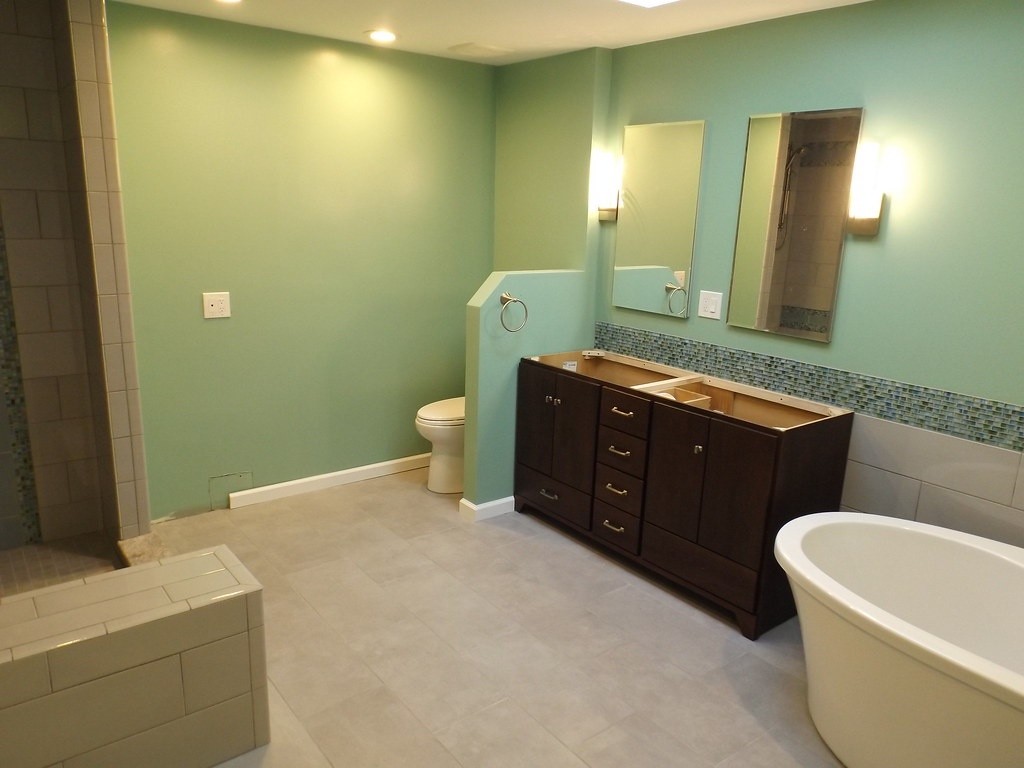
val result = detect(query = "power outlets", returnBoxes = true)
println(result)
[673,270,686,287]
[698,290,724,320]
[203,291,232,318]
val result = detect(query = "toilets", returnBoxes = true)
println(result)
[414,397,465,494]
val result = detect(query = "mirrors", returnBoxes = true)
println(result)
[610,119,706,320]
[726,106,864,344]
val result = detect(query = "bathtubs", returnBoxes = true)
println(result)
[772,511,1024,768]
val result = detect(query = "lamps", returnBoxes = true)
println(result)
[597,202,616,222]
[846,193,885,236]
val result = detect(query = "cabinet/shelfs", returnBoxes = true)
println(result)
[513,349,855,640]
